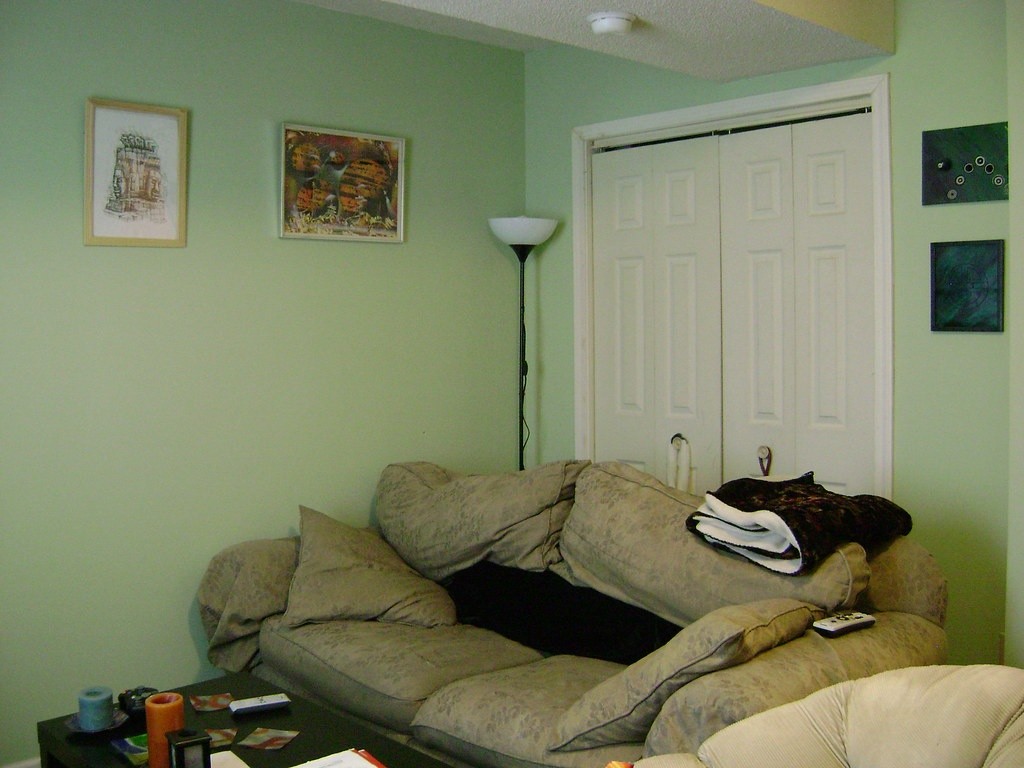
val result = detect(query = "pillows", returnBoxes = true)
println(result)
[281,504,456,628]
[545,597,826,752]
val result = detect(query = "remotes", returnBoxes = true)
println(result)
[229,693,292,715]
[812,612,876,639]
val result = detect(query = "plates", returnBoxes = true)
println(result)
[63,708,130,734]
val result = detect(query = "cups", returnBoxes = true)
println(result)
[145,692,184,768]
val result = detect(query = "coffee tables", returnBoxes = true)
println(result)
[37,672,449,768]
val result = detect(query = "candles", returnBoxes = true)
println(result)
[77,685,113,731]
[145,692,185,768]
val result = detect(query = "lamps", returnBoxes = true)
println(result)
[487,214,558,468]
[586,11,636,35]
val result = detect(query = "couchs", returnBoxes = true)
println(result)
[194,460,949,768]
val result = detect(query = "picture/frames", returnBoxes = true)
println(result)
[930,239,1005,332]
[82,97,188,246]
[279,122,406,244]
